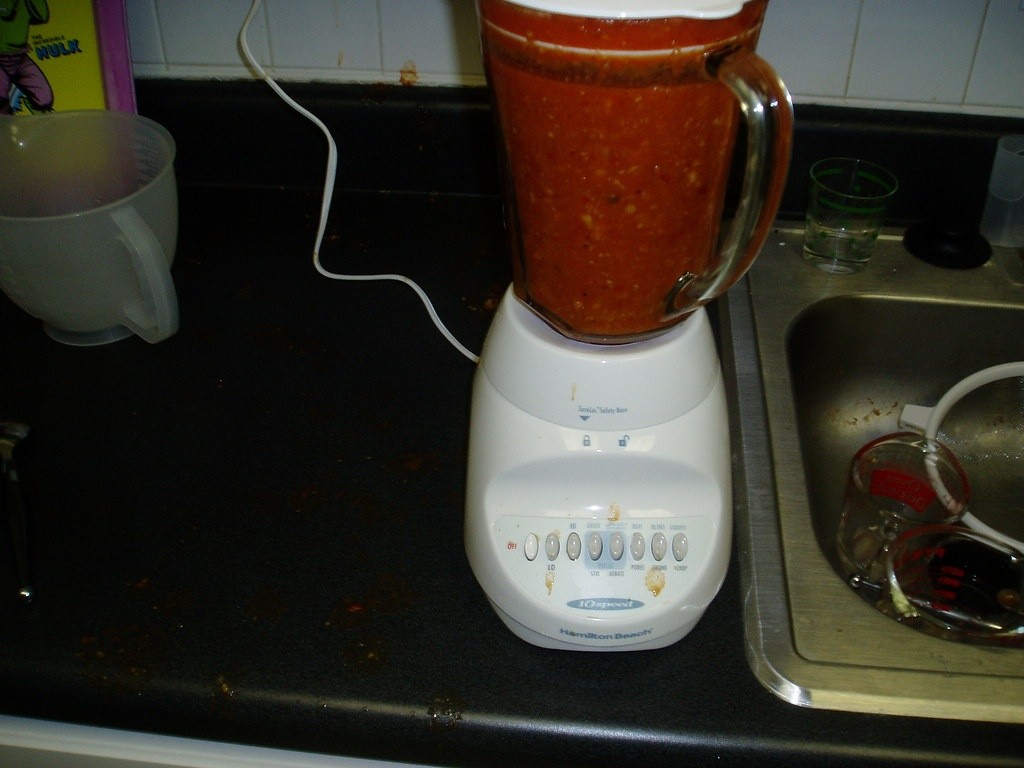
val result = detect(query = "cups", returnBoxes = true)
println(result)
[0,107,179,346]
[803,158,898,274]
[856,524,1024,650]
[835,432,972,591]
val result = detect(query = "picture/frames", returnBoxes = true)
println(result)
[0,0,140,208]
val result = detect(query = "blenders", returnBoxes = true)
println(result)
[464,0,793,652]
